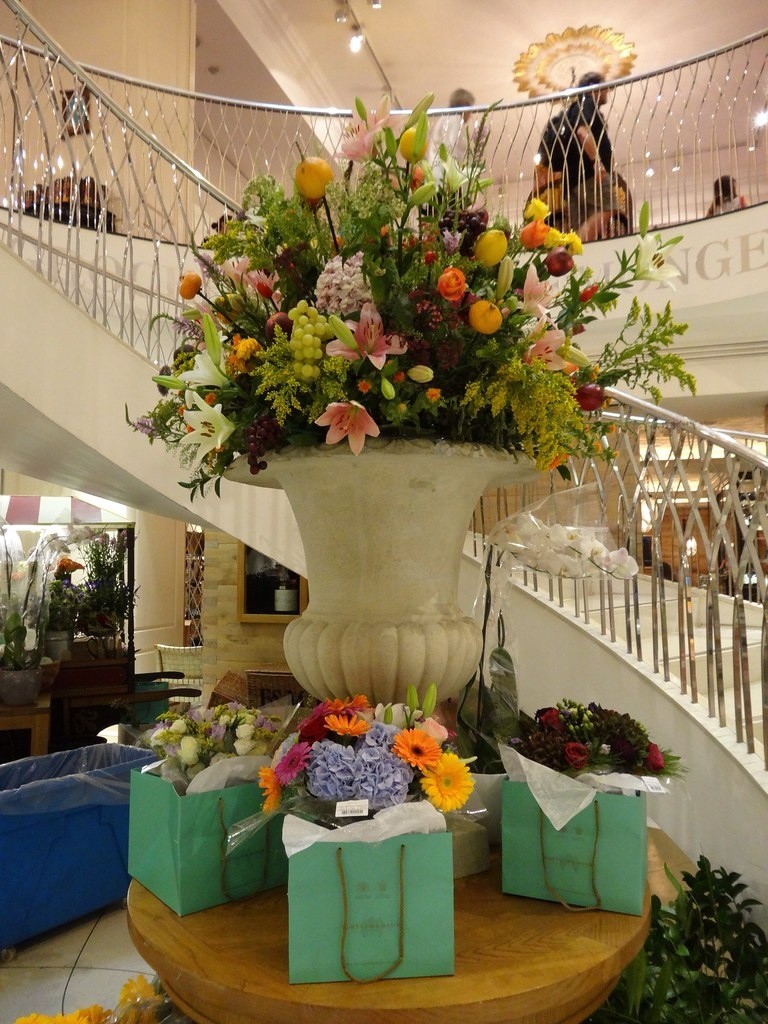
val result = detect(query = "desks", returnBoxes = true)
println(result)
[120,853,653,1024]
[0,692,52,757]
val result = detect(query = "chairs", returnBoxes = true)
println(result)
[155,643,205,713]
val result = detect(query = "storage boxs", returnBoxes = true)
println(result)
[0,742,159,962]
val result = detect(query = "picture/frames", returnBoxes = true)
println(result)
[59,87,91,139]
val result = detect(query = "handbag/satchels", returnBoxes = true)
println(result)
[127,758,290,918]
[497,769,647,918]
[286,794,454,985]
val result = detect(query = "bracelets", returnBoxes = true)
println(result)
[592,158,601,162]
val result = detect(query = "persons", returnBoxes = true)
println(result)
[706,175,748,218]
[426,88,478,200]
[540,71,619,244]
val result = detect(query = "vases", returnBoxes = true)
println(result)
[448,823,492,881]
[86,633,124,660]
[1,666,42,708]
[453,772,509,835]
[45,633,74,665]
[218,437,540,721]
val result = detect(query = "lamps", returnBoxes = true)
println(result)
[335,3,351,24]
[349,24,366,52]
[372,0,382,9]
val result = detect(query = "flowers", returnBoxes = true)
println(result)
[120,93,700,500]
[0,517,104,671]
[12,974,165,1024]
[255,682,476,818]
[81,527,144,635]
[138,702,288,786]
[40,556,85,633]
[505,698,692,784]
[449,512,639,773]
